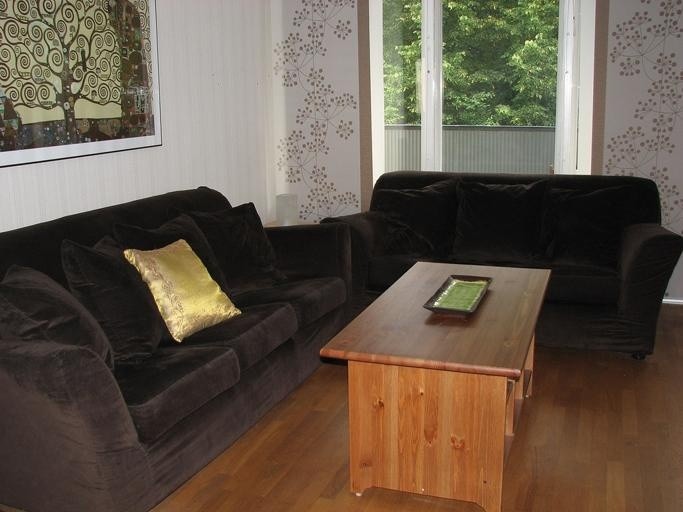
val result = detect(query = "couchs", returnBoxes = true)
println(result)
[1,186,352,512]
[320,171,683,360]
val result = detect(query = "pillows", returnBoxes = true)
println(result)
[372,179,632,267]
[114,214,229,294]
[123,239,242,342]
[61,235,164,360]
[182,202,281,280]
[2,263,114,369]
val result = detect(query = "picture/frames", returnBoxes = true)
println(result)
[0,0,163,169]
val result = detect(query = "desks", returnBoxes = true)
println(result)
[320,263,552,511]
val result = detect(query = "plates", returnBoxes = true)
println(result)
[422,274,493,319]
